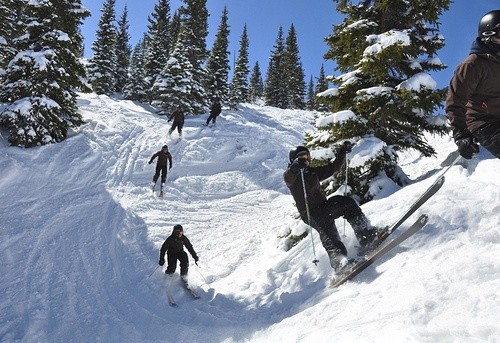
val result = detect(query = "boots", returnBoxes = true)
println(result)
[360,227,382,245]
[202,122,208,126]
[180,278,188,286]
[212,123,215,126]
[161,183,165,188]
[152,182,155,187]
[333,255,357,276]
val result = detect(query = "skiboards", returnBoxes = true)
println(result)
[329,176,445,289]
[165,138,182,144]
[169,285,200,304]
[153,187,163,199]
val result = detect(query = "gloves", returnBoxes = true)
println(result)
[194,256,198,261]
[148,161,151,164]
[159,260,164,266]
[341,141,351,153]
[290,157,307,173]
[456,134,480,160]
[167,119,171,123]
[169,166,172,168]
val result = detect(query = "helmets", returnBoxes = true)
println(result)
[478,11,500,38]
[214,97,219,101]
[174,225,183,232]
[162,145,168,150]
[289,146,309,160]
[176,105,181,108]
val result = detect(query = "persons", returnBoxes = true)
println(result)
[159,222,201,293]
[148,143,173,190]
[204,93,222,127]
[443,8,500,161]
[282,139,388,279]
[167,102,184,134]
